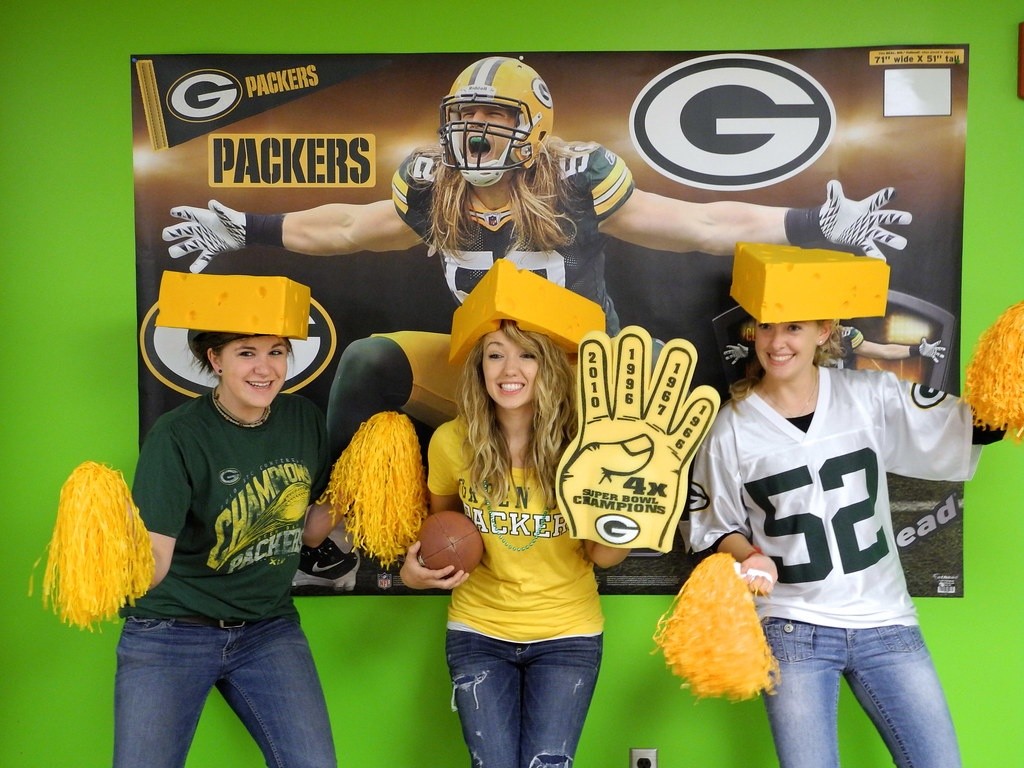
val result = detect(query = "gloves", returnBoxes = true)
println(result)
[723,343,748,365]
[909,337,946,364]
[162,199,285,274]
[783,180,912,263]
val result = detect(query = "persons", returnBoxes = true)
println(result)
[724,318,948,371]
[689,292,1024,768]
[160,57,914,592]
[400,305,636,768]
[110,319,381,768]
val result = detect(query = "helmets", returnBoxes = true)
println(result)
[437,56,554,171]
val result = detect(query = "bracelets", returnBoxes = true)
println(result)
[746,346,757,357]
[908,344,920,356]
[746,546,766,559]
[783,203,825,247]
[244,210,288,251]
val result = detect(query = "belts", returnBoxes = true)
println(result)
[174,616,252,628]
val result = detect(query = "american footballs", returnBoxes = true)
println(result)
[415,511,484,578]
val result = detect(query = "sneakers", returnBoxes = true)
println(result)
[291,536,360,591]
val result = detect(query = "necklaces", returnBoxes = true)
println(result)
[760,367,818,417]
[212,387,271,428]
[481,477,549,552]
